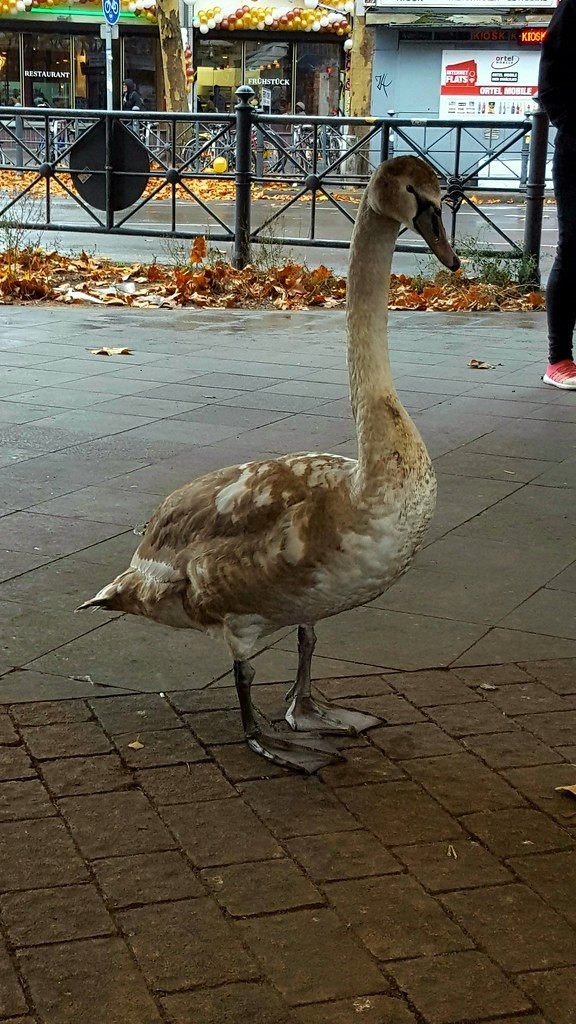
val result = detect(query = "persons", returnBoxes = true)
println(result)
[296,101,306,115]
[1,86,51,109]
[538,0,576,391]
[197,85,225,113]
[122,79,144,111]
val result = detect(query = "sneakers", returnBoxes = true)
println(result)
[543,359,576,390]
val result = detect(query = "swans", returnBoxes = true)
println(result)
[72,153,462,781]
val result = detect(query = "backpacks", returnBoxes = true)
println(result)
[128,91,147,110]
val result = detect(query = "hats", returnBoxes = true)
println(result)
[296,102,306,111]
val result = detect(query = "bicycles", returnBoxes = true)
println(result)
[141,121,342,179]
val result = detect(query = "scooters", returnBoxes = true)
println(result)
[38,102,68,166]
